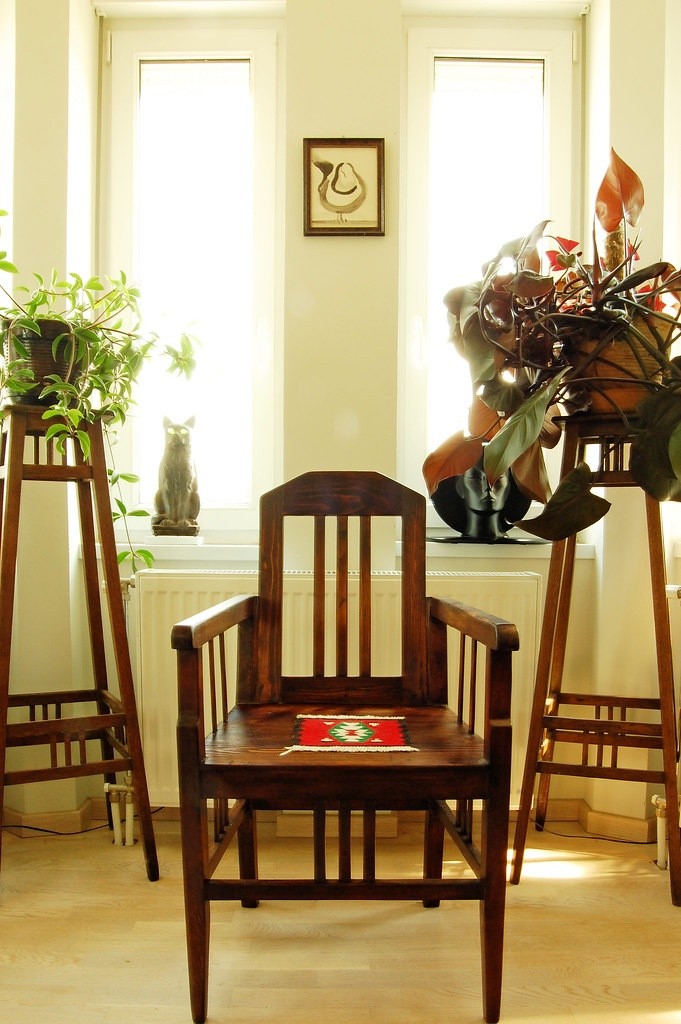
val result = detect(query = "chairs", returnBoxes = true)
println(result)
[170,469,521,1024]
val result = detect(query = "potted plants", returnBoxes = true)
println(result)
[0,249,195,575]
[421,146,681,542]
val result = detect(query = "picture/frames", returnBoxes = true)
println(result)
[302,137,384,237]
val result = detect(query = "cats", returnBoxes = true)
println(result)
[152,416,202,527]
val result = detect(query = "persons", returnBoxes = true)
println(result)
[455,459,512,537]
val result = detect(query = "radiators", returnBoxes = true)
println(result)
[134,567,542,811]
